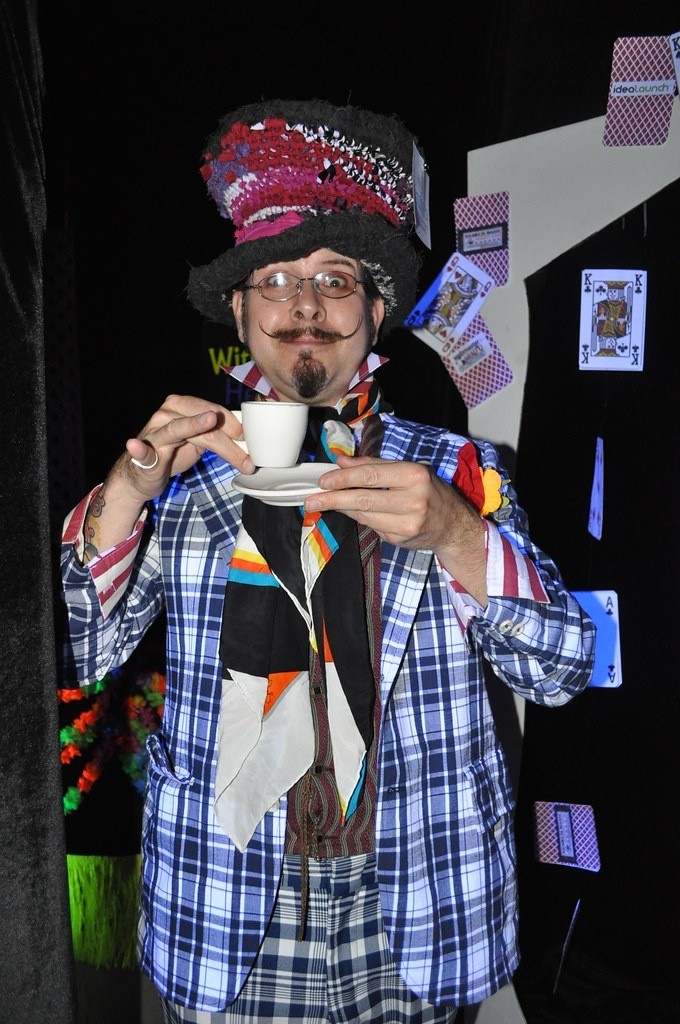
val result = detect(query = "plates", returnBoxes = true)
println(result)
[231,463,341,506]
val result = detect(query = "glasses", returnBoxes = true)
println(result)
[236,270,368,302]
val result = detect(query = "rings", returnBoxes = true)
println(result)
[131,448,160,470]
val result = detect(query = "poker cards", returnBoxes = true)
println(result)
[408,30,679,877]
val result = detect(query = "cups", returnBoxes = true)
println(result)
[230,400,309,467]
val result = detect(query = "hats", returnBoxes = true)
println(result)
[186,98,428,327]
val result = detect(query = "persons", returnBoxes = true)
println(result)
[61,104,596,1021]
